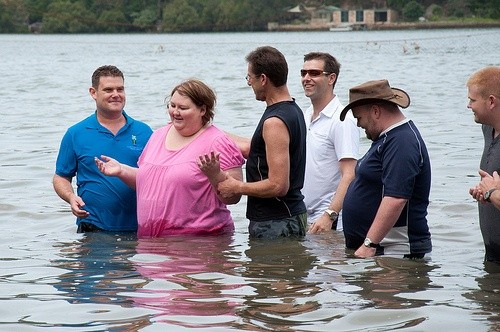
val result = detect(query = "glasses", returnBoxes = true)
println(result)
[245,75,261,82]
[301,70,331,77]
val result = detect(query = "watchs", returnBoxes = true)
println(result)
[484,187,498,203]
[363,238,380,248]
[324,209,338,220]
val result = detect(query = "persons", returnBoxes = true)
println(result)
[93,78,245,238]
[468,168,500,211]
[217,46,307,240]
[301,51,360,236]
[466,64,500,261]
[339,80,431,257]
[52,64,153,234]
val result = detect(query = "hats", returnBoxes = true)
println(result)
[339,79,411,122]
